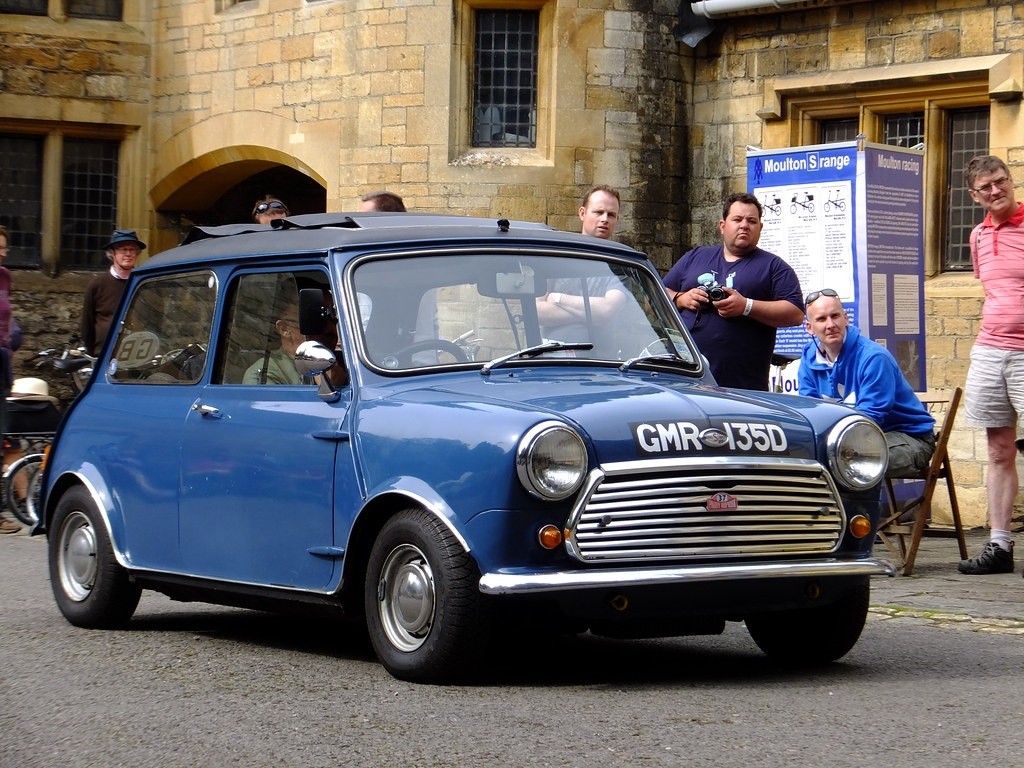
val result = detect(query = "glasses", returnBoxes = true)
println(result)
[253,201,288,219]
[805,289,839,311]
[973,175,1009,194]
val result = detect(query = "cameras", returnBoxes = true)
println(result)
[705,284,730,302]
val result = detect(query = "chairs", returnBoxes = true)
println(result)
[875,387,968,576]
[180,349,266,383]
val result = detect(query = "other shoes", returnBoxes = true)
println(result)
[0,515,21,534]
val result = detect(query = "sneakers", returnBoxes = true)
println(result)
[958,540,1014,573]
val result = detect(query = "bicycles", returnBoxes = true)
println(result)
[0,345,208,525]
[438,331,483,363]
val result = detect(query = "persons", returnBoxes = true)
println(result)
[957,155,1024,574]
[663,192,803,390]
[798,290,936,512]
[1,228,22,533]
[360,190,407,213]
[241,278,350,385]
[251,196,288,225]
[533,184,630,360]
[81,230,165,364]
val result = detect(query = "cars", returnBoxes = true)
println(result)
[30,213,890,686]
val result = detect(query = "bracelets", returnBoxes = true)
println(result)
[743,298,753,316]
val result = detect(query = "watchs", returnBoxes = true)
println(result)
[554,293,562,306]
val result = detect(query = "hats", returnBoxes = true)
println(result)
[104,230,147,250]
[6,377,59,404]
